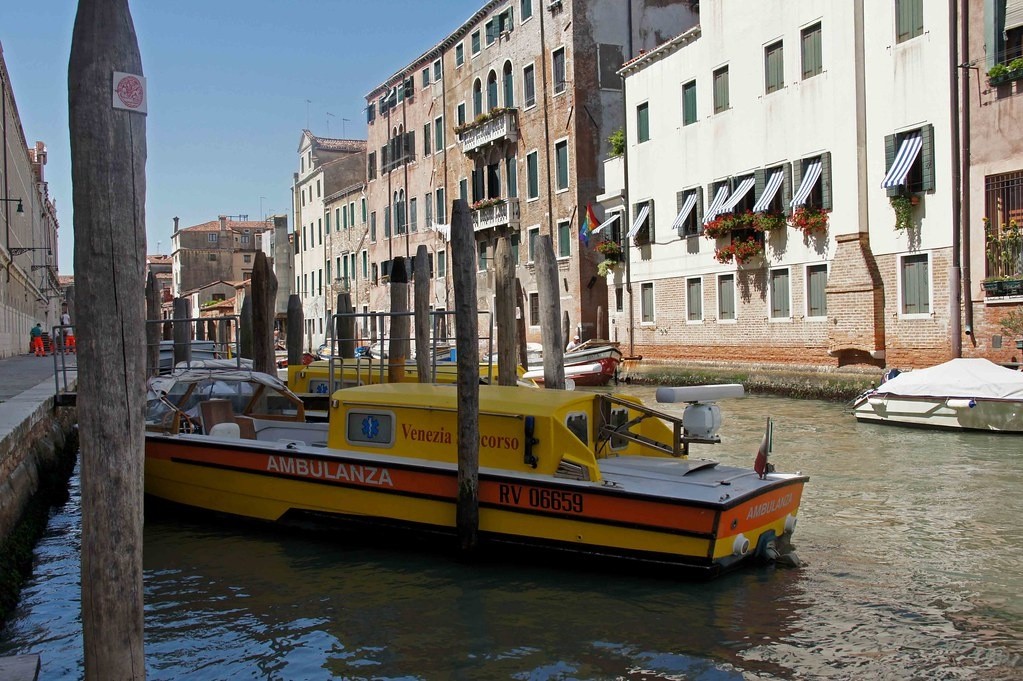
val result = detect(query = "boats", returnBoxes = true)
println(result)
[523,337,643,386]
[850,358,1023,433]
[160,334,541,393]
[145,311,810,576]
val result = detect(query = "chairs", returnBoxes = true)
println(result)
[197,398,256,439]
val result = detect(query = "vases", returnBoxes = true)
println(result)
[983,279,1023,297]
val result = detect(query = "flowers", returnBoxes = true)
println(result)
[594,235,622,253]
[981,217,1023,282]
[452,106,510,135]
[704,204,829,265]
[468,196,507,211]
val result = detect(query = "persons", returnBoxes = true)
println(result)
[566,335,582,353]
[60,310,71,325]
[63,327,76,355]
[47,326,59,355]
[273,327,279,345]
[29,323,48,357]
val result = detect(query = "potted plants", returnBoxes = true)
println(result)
[889,191,921,239]
[989,57,1023,86]
[597,258,617,277]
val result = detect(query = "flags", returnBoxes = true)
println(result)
[579,201,600,249]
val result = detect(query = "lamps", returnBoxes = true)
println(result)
[47,296,66,305]
[8,247,53,257]
[39,286,63,295]
[0,197,24,216]
[31,265,58,272]
[545,1,562,11]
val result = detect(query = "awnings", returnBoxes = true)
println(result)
[880,128,922,189]
[625,204,650,238]
[702,182,729,224]
[789,156,822,207]
[671,190,697,230]
[715,175,755,215]
[591,214,620,235]
[753,168,784,213]
